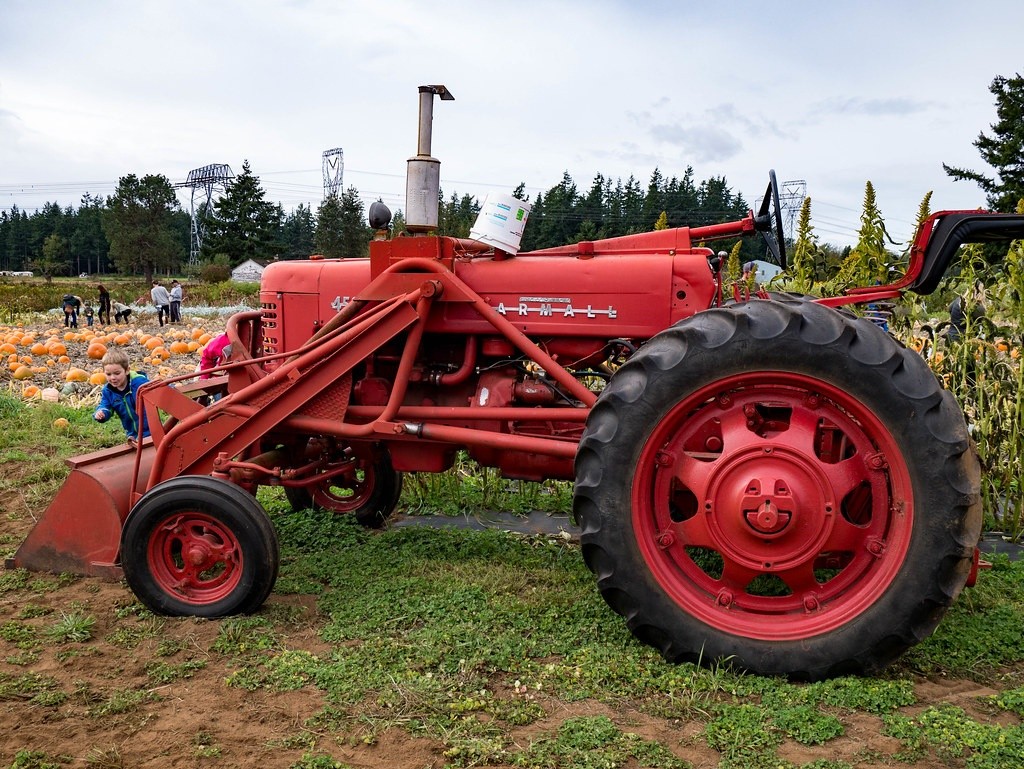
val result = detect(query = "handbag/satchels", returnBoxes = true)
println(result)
[65,305,73,314]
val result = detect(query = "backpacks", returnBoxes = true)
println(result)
[63,295,74,301]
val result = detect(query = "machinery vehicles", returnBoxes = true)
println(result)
[2,84,1024,686]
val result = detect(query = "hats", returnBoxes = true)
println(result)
[172,280,177,284]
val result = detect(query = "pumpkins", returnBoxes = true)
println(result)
[909,335,1023,382]
[0,321,223,428]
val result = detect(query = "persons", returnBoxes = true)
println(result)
[170,280,182,325]
[151,280,170,326]
[111,300,132,324]
[83,300,95,326]
[93,349,183,439]
[62,294,82,329]
[96,285,111,325]
[195,328,232,402]
